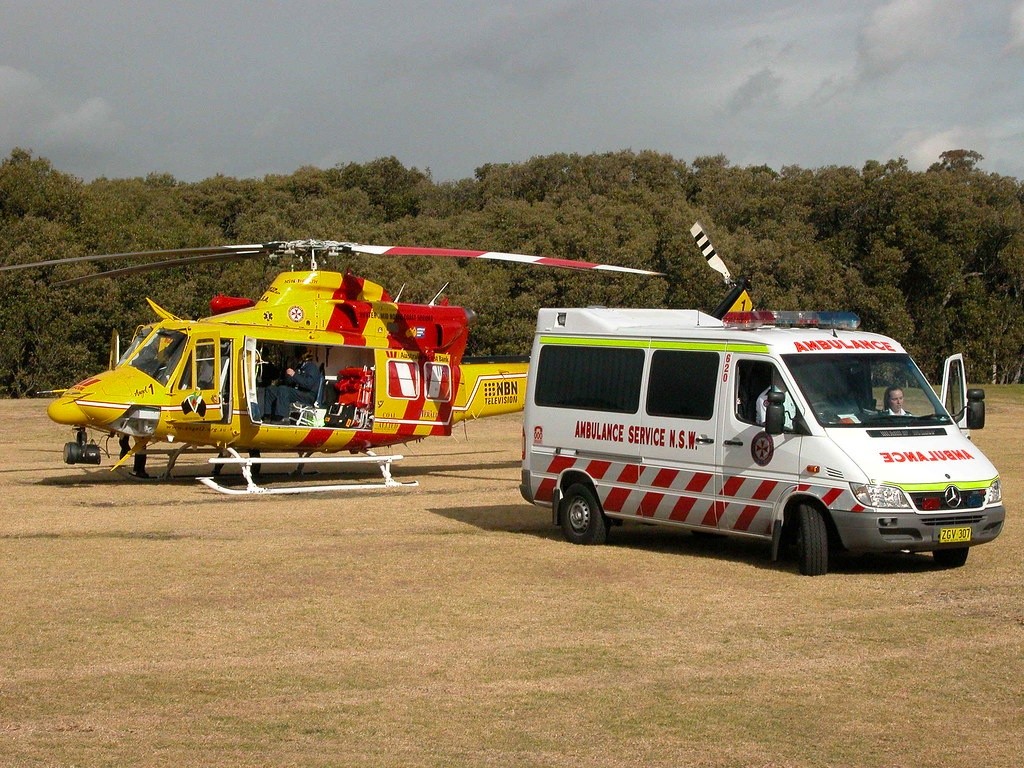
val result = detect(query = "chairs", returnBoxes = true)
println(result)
[288,368,325,426]
[207,342,229,396]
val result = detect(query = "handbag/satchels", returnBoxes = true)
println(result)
[324,403,355,427]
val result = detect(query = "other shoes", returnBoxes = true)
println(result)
[270,417,291,425]
[120,448,130,460]
[136,470,149,478]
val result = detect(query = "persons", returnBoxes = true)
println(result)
[132,347,166,384]
[755,371,798,429]
[259,346,320,424]
[879,387,915,416]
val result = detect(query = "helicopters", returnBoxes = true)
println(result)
[1,222,757,496]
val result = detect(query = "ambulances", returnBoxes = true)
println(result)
[519,303,1006,577]
